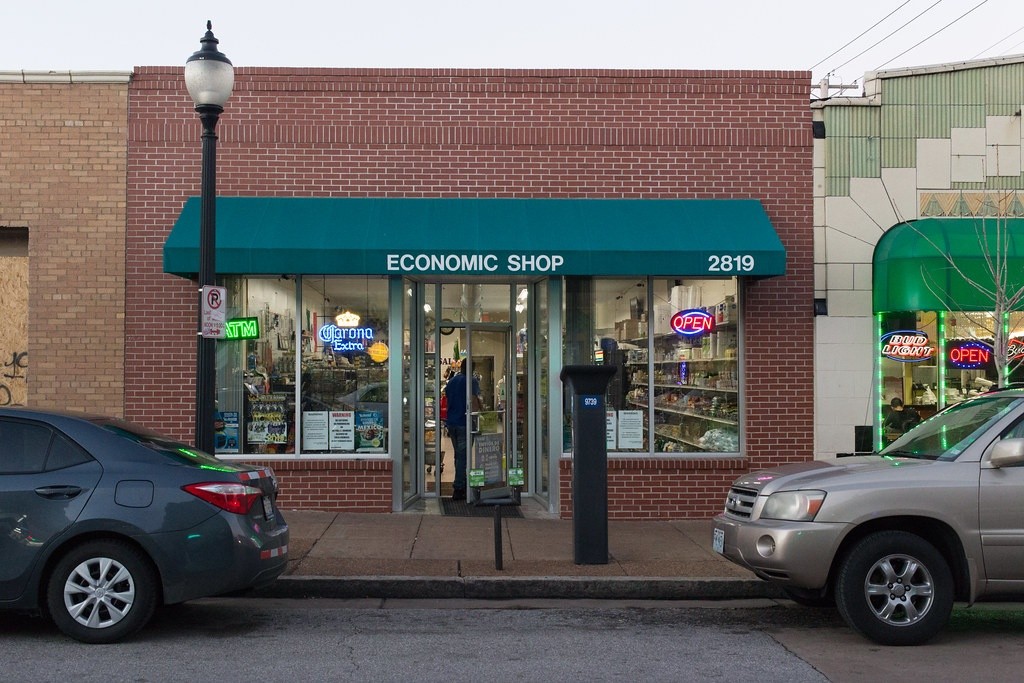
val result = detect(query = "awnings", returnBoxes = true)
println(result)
[163,195,787,278]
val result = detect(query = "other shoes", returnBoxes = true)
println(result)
[453,489,468,500]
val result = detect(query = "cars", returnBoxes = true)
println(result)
[0,406,290,644]
[710,387,1024,647]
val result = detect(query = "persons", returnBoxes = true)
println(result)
[886,396,925,434]
[444,358,488,500]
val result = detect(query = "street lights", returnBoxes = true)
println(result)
[185,19,234,457]
